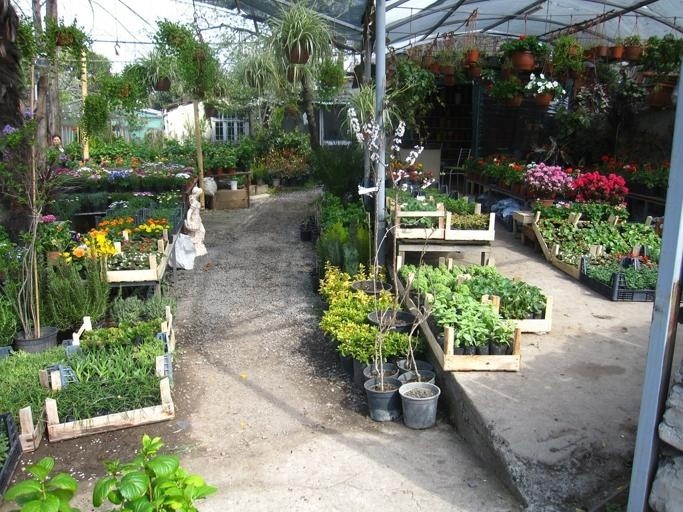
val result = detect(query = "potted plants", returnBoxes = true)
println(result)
[204,156,237,174]
[553,36,582,70]
[585,34,681,78]
[420,39,490,90]
[41,15,92,79]
[317,255,443,430]
[83,0,345,142]
[493,77,523,107]
[396,256,548,371]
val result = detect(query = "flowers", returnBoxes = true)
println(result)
[526,73,567,98]
[349,108,426,328]
[72,156,191,268]
[466,154,630,201]
[0,110,70,334]
[500,34,547,56]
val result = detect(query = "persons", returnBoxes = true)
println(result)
[49,135,65,155]
[184,187,208,257]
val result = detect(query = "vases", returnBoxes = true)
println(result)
[535,93,553,107]
[11,327,59,352]
[511,50,536,71]
